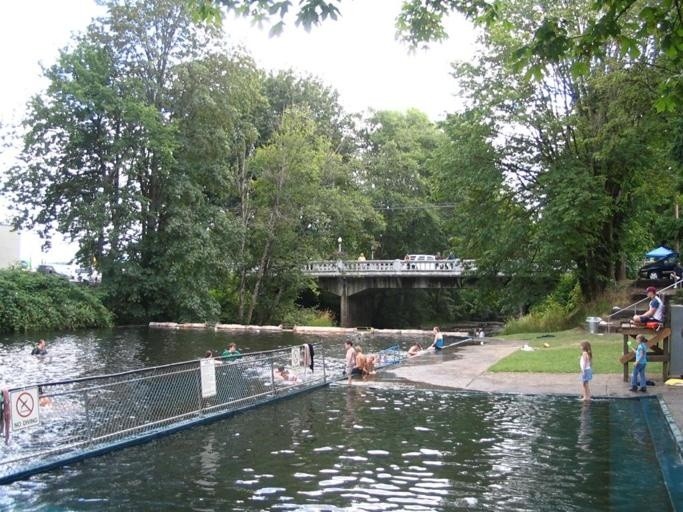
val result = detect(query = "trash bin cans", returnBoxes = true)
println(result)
[585,317,599,335]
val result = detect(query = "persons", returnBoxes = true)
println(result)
[580,340,594,401]
[203,349,221,366]
[435,251,441,269]
[31,339,49,356]
[37,381,50,408]
[223,343,244,365]
[278,364,301,383]
[345,340,387,385]
[358,252,367,269]
[427,326,443,350]
[650,271,658,280]
[670,272,676,281]
[479,327,485,345]
[633,287,664,323]
[447,249,455,269]
[405,253,410,269]
[408,341,422,358]
[629,334,648,393]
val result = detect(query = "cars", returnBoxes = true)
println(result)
[637,262,673,279]
[19,255,101,285]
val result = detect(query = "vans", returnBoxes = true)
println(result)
[400,253,443,270]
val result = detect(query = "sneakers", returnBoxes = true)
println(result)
[629,386,638,391]
[639,387,646,391]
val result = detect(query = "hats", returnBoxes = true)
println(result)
[643,287,656,293]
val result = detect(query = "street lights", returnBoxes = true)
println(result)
[337,236,342,254]
[369,244,378,261]
